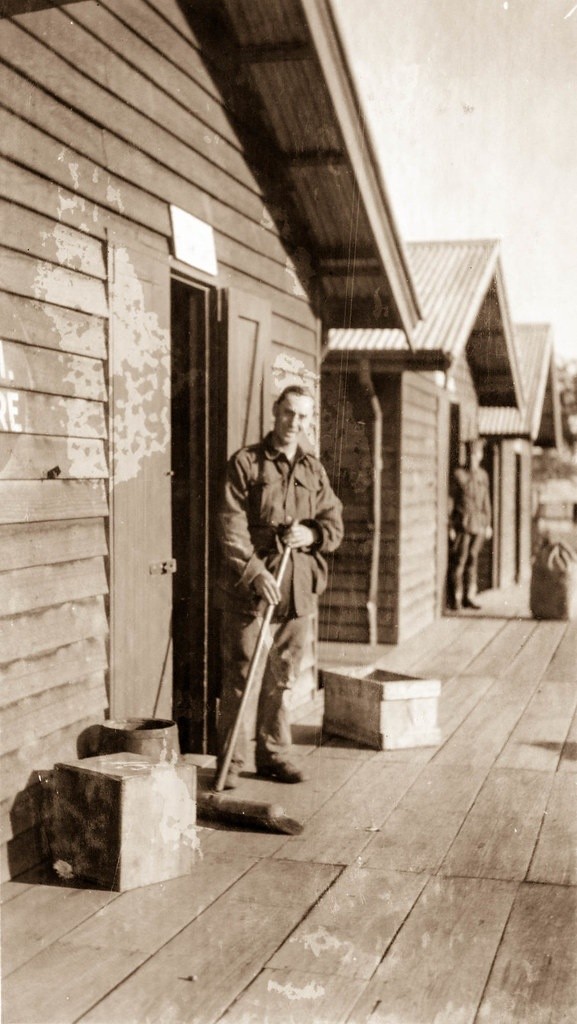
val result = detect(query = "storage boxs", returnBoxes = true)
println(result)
[54,751,197,894]
[322,665,439,748]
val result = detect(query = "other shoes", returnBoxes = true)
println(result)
[256,761,302,783]
[462,599,481,609]
[447,601,461,610]
[215,758,244,788]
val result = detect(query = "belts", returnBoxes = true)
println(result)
[254,542,315,559]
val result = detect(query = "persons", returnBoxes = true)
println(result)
[212,382,344,789]
[442,438,494,610]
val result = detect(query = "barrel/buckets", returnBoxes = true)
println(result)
[101,717,181,768]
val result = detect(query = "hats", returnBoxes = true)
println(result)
[465,439,485,451]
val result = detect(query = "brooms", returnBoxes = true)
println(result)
[191,518,304,835]
